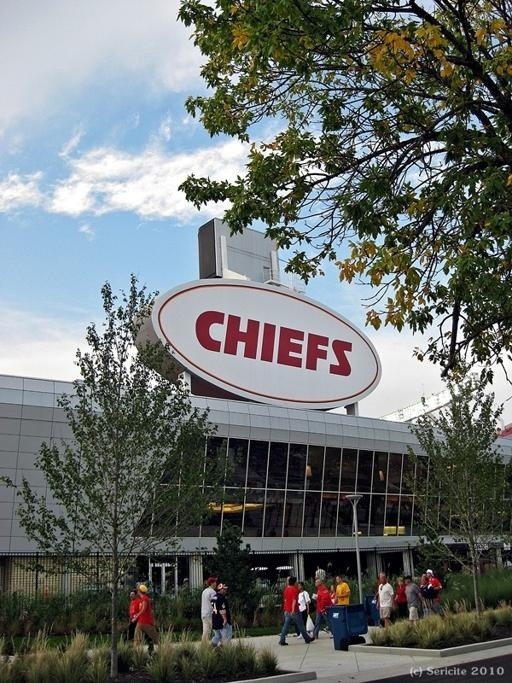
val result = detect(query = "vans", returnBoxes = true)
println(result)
[247,564,295,590]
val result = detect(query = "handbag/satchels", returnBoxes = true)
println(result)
[211,614,232,630]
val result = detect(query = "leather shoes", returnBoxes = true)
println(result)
[307,637,315,644]
[280,642,288,645]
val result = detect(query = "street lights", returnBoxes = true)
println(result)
[343,490,368,607]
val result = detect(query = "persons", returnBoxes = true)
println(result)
[210,582,234,647]
[276,569,444,647]
[128,584,162,655]
[200,577,219,645]
[123,591,155,655]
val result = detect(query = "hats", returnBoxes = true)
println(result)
[208,576,218,585]
[402,575,412,579]
[217,583,228,590]
[427,569,435,577]
[139,585,148,594]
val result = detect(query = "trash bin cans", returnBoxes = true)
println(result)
[324,603,368,651]
[366,594,381,626]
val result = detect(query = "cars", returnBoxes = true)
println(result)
[504,559,512,568]
